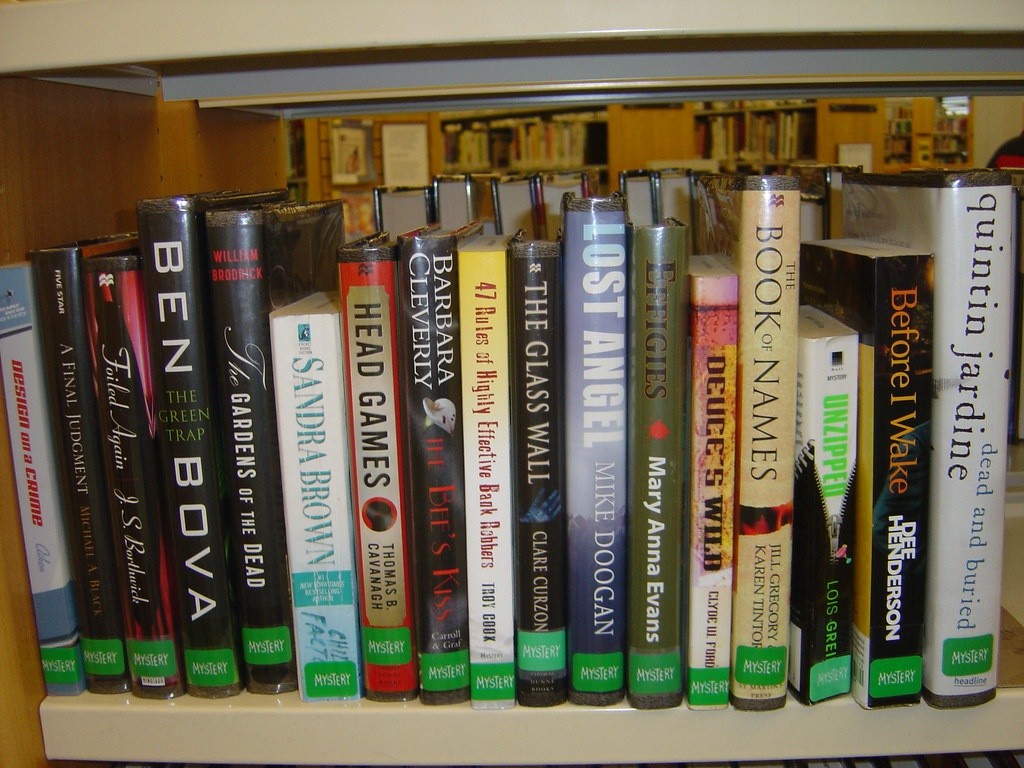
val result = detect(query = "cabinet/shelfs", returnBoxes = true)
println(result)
[0,0,1023,768]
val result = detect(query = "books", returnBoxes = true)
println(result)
[443,116,607,168]
[692,99,969,170]
[1,167,1024,712]
